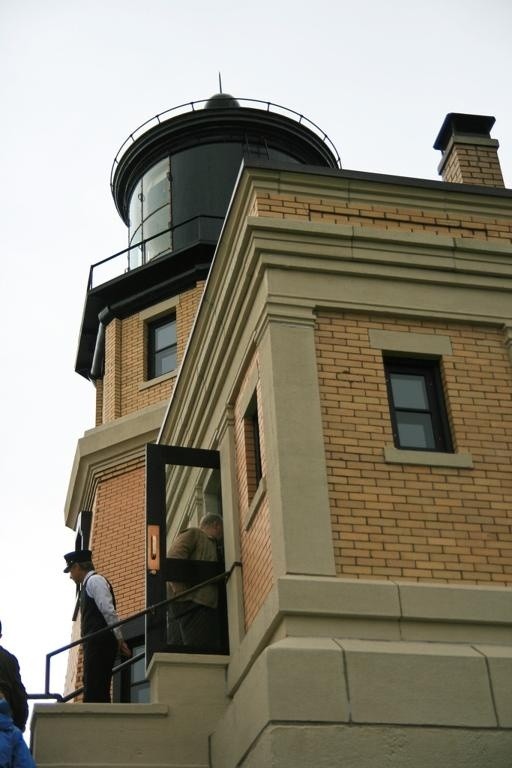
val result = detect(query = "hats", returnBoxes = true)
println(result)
[64,551,92,572]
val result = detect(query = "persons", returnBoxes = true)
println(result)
[160,512,224,649]
[60,546,135,708]
[0,617,30,731]
[1,687,36,767]
